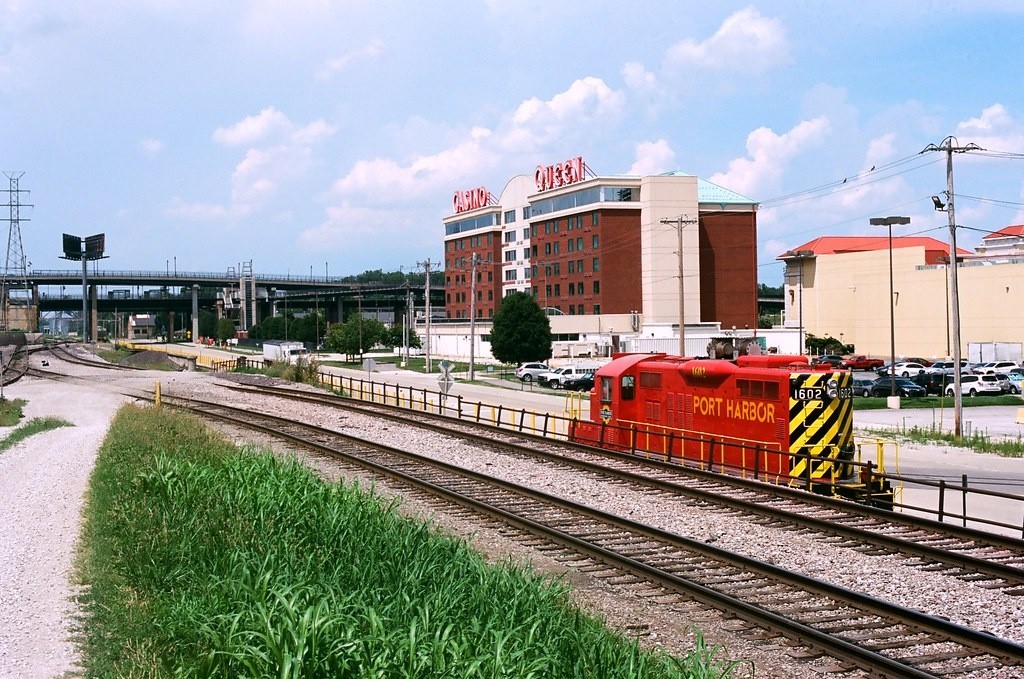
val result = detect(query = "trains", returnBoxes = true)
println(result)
[565,334,909,514]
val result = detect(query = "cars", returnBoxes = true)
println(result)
[872,377,928,399]
[1009,367,1024,377]
[946,374,1002,398]
[853,379,879,398]
[564,372,596,392]
[812,356,847,368]
[877,361,902,373]
[901,356,934,368]
[886,362,933,379]
[514,362,555,383]
[988,372,1024,394]
[907,373,958,397]
[970,362,987,375]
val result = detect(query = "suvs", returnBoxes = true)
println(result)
[973,361,1020,376]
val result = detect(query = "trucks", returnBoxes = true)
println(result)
[263,338,311,370]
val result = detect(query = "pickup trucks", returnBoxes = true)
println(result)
[837,355,885,372]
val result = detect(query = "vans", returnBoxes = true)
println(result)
[927,361,972,377]
[538,364,602,390]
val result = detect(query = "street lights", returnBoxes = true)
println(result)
[869,216,911,398]
[786,249,816,355]
